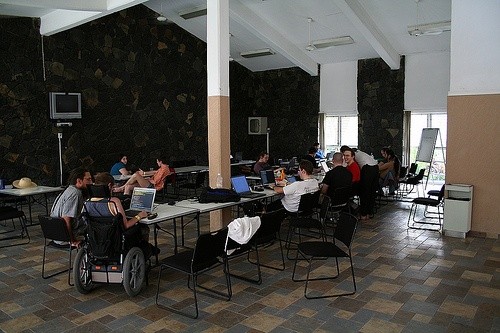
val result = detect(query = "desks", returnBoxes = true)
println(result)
[166,189,284,246]
[113,166,209,181]
[246,175,262,180]
[0,186,65,227]
[230,160,256,166]
[126,204,200,286]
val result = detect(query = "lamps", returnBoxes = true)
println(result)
[306,17,315,51]
[178,5,207,20]
[313,36,354,48]
[156,0,166,22]
[408,21,451,36]
[409,0,423,37]
[240,48,274,58]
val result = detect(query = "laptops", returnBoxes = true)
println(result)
[320,160,330,173]
[233,152,243,161]
[318,151,335,167]
[259,170,285,191]
[124,187,156,219]
[231,175,266,199]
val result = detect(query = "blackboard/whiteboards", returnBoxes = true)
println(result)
[416,128,439,163]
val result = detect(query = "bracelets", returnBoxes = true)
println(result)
[135,215,141,220]
[272,186,276,188]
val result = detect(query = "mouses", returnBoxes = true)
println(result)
[148,215,155,220]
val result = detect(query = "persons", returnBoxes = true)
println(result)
[112,156,172,203]
[81,172,160,261]
[111,154,132,175]
[264,161,320,220]
[48,167,93,248]
[306,142,402,221]
[255,152,284,173]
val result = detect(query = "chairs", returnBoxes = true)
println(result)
[38,215,86,287]
[156,152,445,318]
[0,179,31,248]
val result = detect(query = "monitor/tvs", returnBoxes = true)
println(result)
[49,93,82,119]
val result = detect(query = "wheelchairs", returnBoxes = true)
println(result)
[70,183,161,297]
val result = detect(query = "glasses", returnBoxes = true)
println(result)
[84,177,91,181]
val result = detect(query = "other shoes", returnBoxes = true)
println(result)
[357,217,367,220]
[388,192,395,195]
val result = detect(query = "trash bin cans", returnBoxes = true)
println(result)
[443,183,475,239]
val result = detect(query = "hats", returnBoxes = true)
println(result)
[12,177,38,188]
